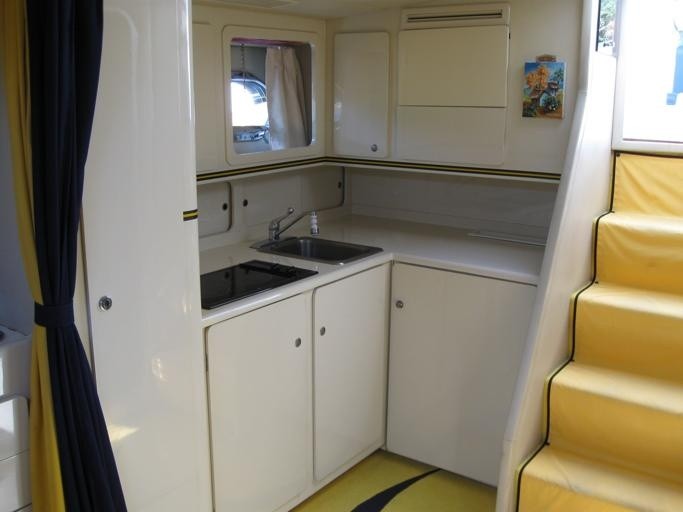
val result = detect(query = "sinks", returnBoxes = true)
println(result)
[249,234,384,266]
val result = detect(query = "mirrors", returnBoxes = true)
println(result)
[229,38,314,153]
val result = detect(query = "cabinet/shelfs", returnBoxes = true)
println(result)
[387,267,536,490]
[206,255,391,511]
[326,18,397,160]
[80,2,214,510]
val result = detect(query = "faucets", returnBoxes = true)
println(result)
[269,206,321,237]
